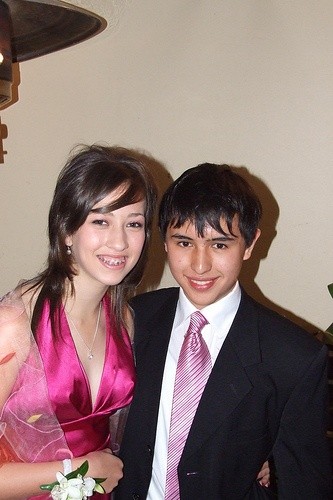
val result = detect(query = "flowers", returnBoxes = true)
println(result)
[40,460,109,500]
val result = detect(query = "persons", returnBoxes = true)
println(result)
[0,143,270,500]
[269,342,332,500]
[111,162,325,500]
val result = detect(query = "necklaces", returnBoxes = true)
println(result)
[62,299,102,360]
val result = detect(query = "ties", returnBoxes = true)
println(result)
[165,311,212,500]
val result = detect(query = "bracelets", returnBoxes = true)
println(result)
[62,459,73,476]
